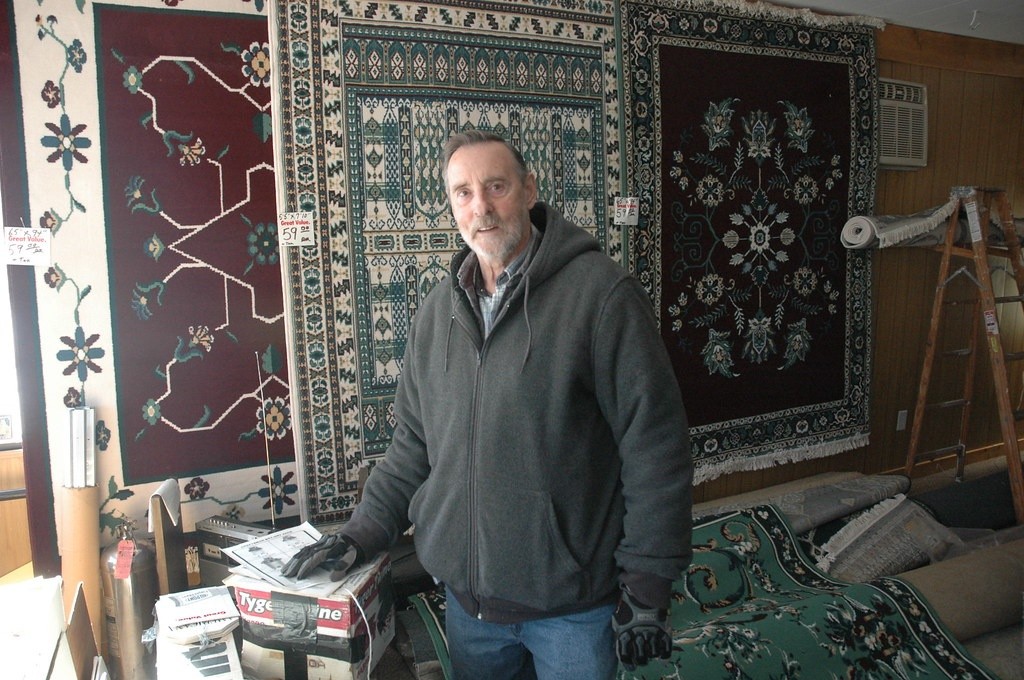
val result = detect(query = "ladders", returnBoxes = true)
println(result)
[904,184,1024,525]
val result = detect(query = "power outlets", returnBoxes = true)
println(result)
[896,410,908,431]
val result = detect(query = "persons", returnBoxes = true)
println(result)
[281,130,694,680]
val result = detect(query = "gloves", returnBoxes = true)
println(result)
[282,532,365,582]
[611,583,672,670]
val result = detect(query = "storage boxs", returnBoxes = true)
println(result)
[233,553,395,680]
[156,629,244,680]
